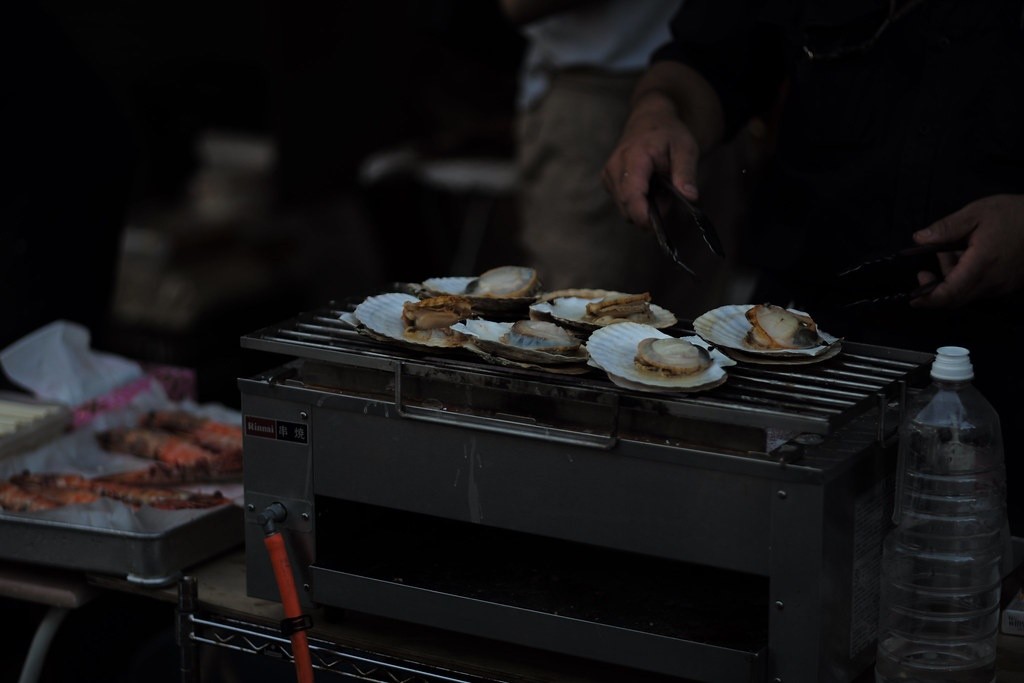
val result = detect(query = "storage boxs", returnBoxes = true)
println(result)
[0,401,246,587]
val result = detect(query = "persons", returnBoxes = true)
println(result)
[605,1,1024,350]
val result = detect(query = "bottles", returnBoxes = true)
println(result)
[871,344,1008,683]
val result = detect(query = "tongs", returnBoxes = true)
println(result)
[642,175,720,276]
[835,236,966,309]
